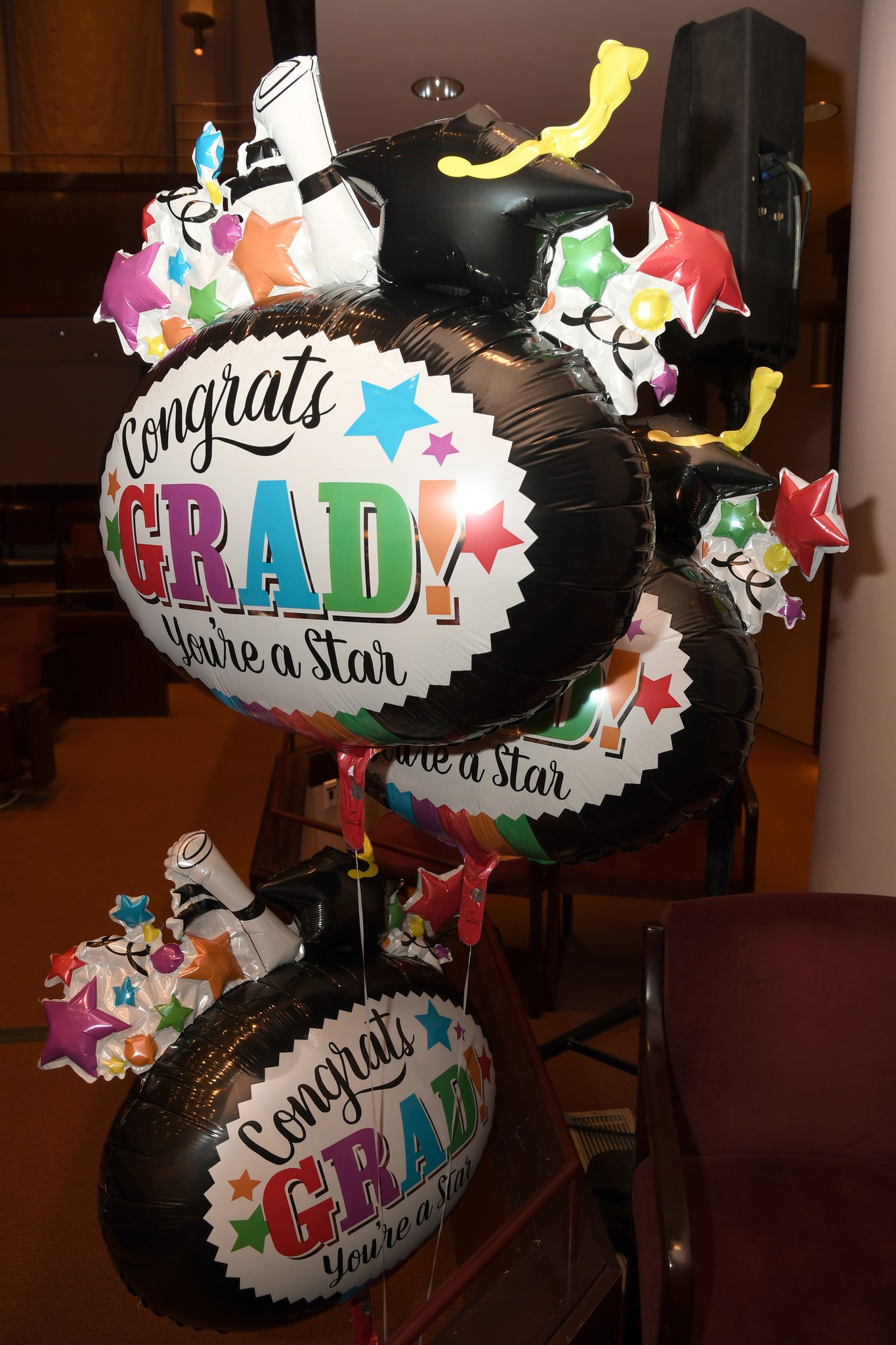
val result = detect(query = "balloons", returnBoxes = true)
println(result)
[36,105,850,1333]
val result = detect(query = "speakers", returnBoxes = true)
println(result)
[655,8,809,375]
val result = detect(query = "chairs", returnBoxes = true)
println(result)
[542,757,761,1009]
[624,888,896,1345]
[360,811,547,1019]
[0,487,170,794]
